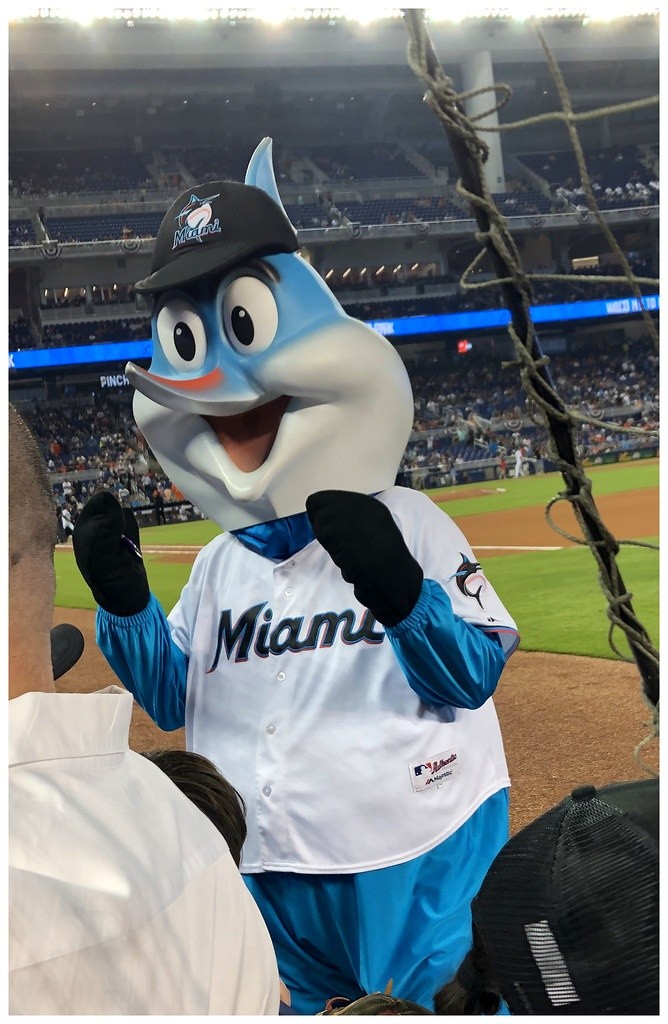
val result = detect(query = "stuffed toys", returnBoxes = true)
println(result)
[77,251,518,1018]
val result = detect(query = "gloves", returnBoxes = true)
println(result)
[305,490,425,628]
[72,490,149,617]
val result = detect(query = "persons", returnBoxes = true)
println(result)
[8,400,288,1015]
[8,136,661,545]
[137,751,246,875]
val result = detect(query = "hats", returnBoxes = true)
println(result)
[465,776,660,1014]
[134,179,299,293]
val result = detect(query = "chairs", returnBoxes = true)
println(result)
[12,143,657,536]
